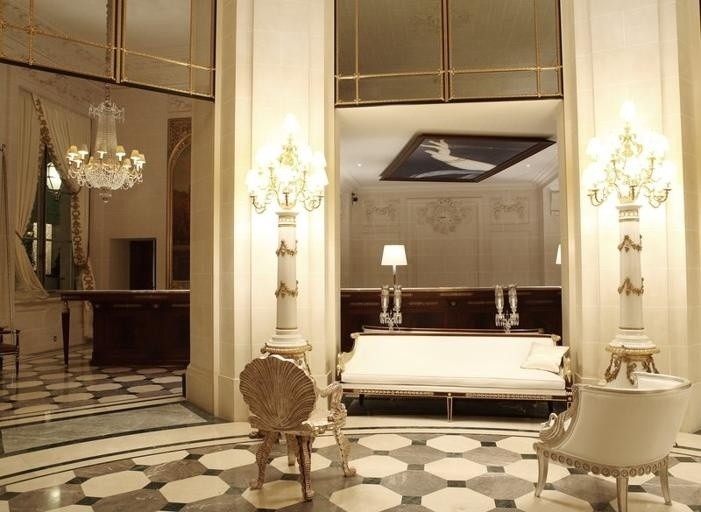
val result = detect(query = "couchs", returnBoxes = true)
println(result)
[335,332,574,423]
[533,371,695,511]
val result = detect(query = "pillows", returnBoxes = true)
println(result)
[519,340,571,374]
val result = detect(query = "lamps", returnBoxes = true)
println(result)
[380,244,411,288]
[45,158,64,202]
[579,108,678,388]
[243,110,332,380]
[64,81,145,203]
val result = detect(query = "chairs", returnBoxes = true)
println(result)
[237,354,355,500]
[0,325,20,372]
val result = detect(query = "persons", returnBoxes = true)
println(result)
[420,139,497,172]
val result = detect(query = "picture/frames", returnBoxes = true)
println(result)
[377,130,556,184]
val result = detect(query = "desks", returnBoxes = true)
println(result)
[53,288,192,371]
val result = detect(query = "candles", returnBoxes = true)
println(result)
[393,287,401,311]
[508,285,519,312]
[380,287,389,312]
[495,285,504,312]
